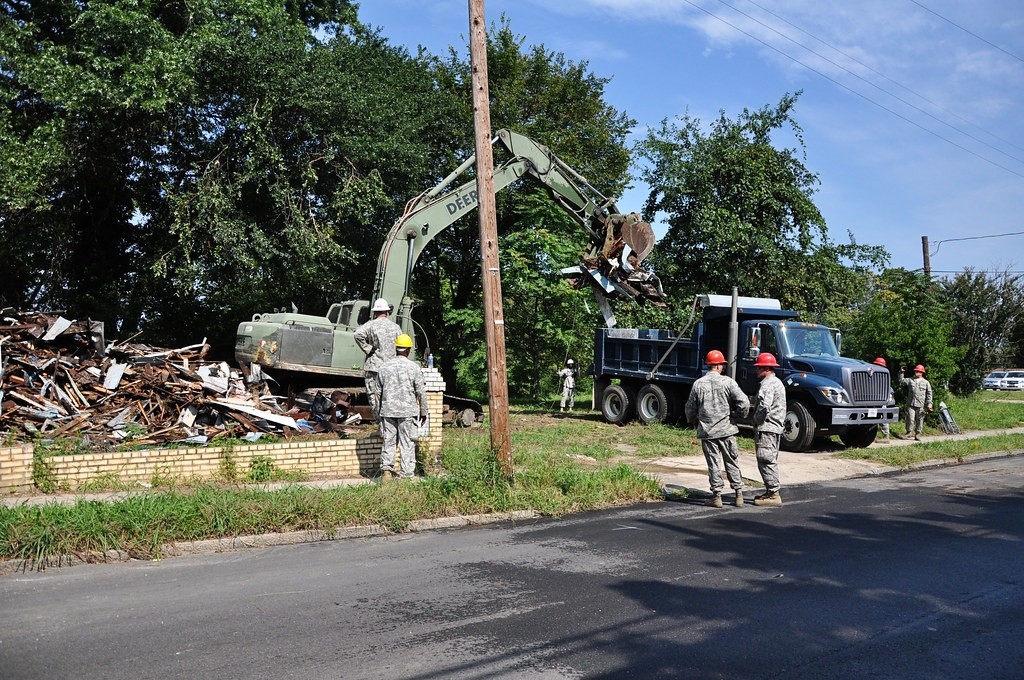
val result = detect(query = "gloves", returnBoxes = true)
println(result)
[900,366,907,374]
[927,404,932,411]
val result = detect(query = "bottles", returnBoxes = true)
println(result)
[428,353,434,368]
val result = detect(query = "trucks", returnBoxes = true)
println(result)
[591,293,901,449]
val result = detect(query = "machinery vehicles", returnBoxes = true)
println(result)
[240,132,667,427]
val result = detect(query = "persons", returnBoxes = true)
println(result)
[873,357,890,443]
[374,334,429,483]
[354,298,403,438]
[685,349,750,507]
[753,352,786,506]
[898,364,932,441]
[558,359,580,413]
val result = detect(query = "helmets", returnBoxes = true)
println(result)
[567,359,574,365]
[914,365,925,373]
[371,298,391,311]
[395,334,413,347]
[705,350,728,364]
[753,353,780,366]
[873,358,886,367]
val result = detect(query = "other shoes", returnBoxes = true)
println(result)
[382,470,393,484]
[569,407,572,411]
[904,431,911,438]
[560,408,563,412]
[915,435,921,441]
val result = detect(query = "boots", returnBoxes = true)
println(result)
[735,496,743,506]
[705,496,723,507]
[754,491,782,506]
[876,435,890,443]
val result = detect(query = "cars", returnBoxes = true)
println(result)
[982,370,1005,392]
[1000,370,1024,391]
